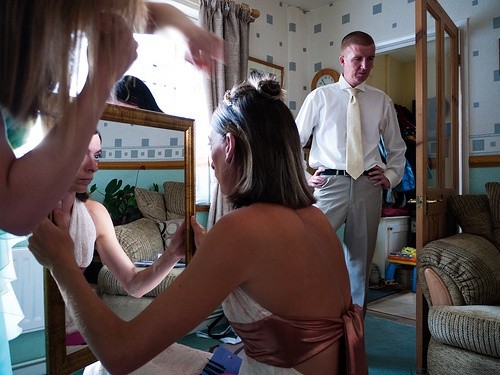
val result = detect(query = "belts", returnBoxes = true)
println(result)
[319,168,379,176]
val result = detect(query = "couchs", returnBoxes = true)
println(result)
[417,181,500,375]
[97,180,181,311]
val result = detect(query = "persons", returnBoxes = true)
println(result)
[28,74,368,375]
[0,1,227,237]
[294,30,406,318]
[57,126,185,354]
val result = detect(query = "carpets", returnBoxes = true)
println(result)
[365,289,418,327]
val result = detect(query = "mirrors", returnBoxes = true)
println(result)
[248,56,284,94]
[43,94,196,375]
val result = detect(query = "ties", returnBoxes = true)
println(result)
[345,88,365,180]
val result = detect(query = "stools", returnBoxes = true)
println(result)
[386,256,423,292]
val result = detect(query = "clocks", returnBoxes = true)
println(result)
[311,68,339,90]
